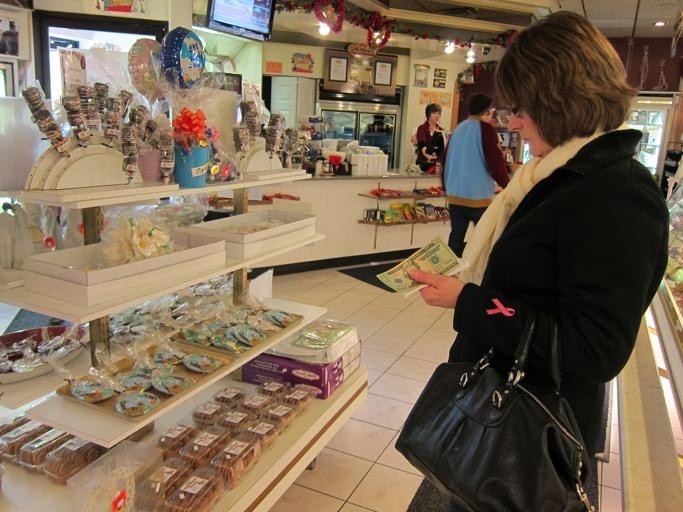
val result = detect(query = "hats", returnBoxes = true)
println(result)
[469,92,491,113]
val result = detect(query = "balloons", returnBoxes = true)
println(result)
[128,27,205,105]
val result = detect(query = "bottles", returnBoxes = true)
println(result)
[367,115,390,132]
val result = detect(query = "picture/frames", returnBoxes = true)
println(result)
[0,4,32,61]
[329,56,347,82]
[374,60,393,85]
[0,57,20,97]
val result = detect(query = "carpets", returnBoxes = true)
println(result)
[337,262,407,293]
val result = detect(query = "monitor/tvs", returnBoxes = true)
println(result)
[206,1,277,41]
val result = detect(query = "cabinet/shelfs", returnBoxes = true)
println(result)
[627,96,676,188]
[358,191,450,226]
[618,186,683,512]
[0,168,367,512]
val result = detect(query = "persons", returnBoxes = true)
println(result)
[411,9,669,512]
[416,103,443,170]
[440,93,510,254]
[360,116,394,158]
[0,20,18,56]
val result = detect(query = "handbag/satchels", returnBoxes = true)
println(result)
[396,308,597,511]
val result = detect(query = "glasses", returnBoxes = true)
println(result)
[505,106,524,120]
